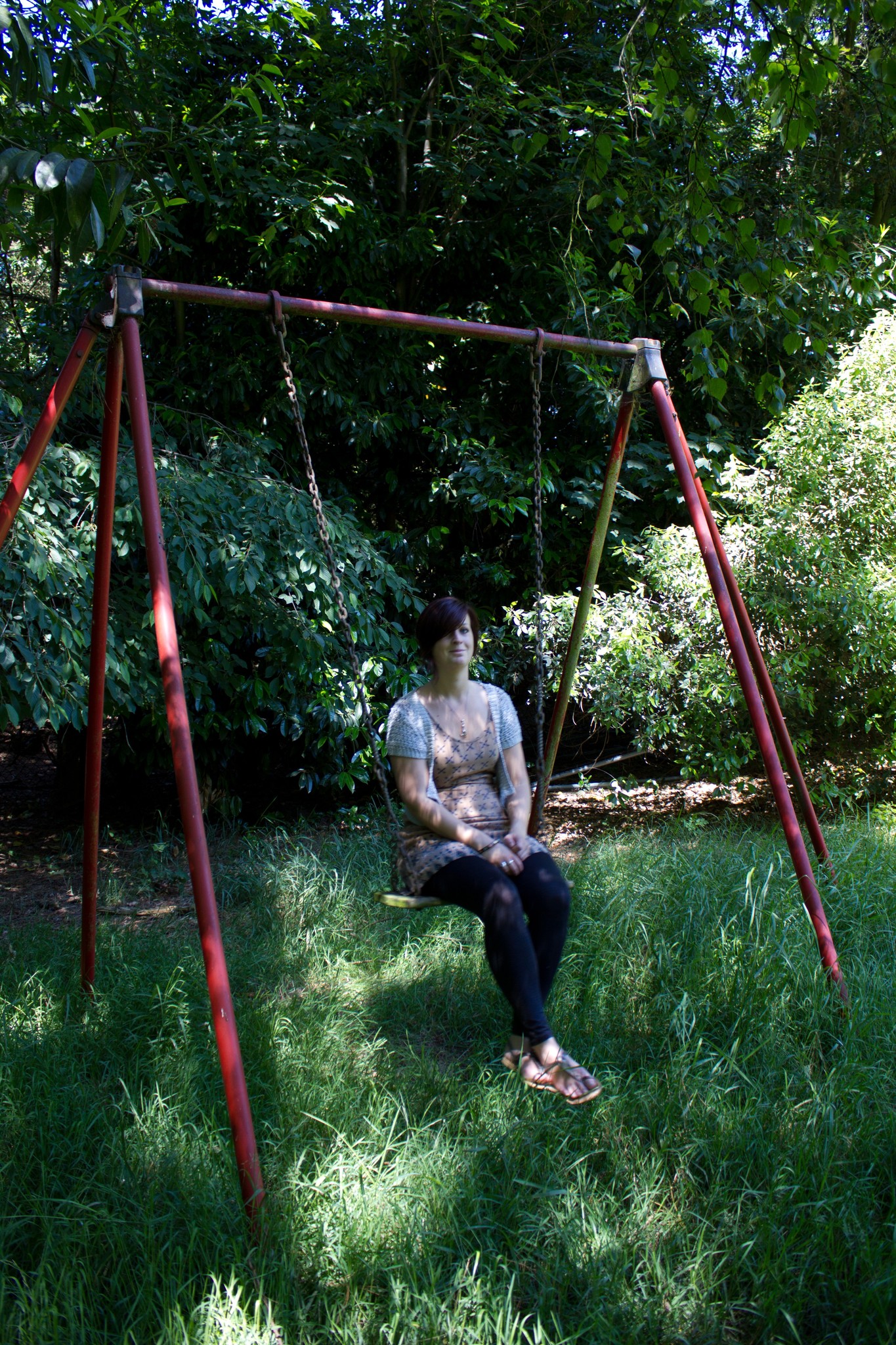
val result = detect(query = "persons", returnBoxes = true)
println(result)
[383,596,603,1106]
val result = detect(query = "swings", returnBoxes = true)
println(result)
[269,323,547,910]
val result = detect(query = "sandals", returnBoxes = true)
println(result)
[542,1051,602,1105]
[502,1040,553,1089]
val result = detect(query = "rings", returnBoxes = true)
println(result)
[507,860,514,865]
[500,862,507,868]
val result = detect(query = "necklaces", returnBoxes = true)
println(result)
[431,680,469,737]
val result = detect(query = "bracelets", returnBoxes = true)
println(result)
[478,838,501,854]
